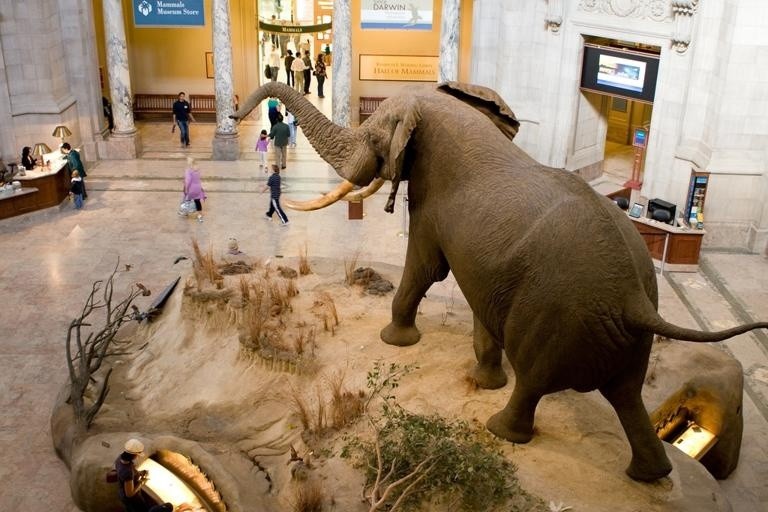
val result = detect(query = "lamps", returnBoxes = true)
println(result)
[34,141,55,170]
[52,124,72,151]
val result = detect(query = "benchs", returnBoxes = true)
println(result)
[131,90,187,123]
[188,92,240,124]
[357,95,386,123]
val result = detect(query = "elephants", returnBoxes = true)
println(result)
[226,79,768,484]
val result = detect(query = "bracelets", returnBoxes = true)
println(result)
[139,480,144,485]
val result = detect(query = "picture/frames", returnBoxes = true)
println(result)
[205,51,215,79]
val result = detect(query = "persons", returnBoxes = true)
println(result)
[105,437,150,511]
[21,145,39,171]
[68,169,85,209]
[177,155,209,223]
[264,164,291,226]
[61,143,88,200]
[256,15,331,172]
[171,91,196,149]
[224,235,248,256]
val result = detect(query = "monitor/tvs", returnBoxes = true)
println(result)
[592,48,650,97]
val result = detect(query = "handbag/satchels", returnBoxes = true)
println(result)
[313,70,316,75]
[264,64,271,78]
[276,112,283,121]
[293,120,299,126]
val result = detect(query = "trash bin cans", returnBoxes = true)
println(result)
[348,199,363,220]
